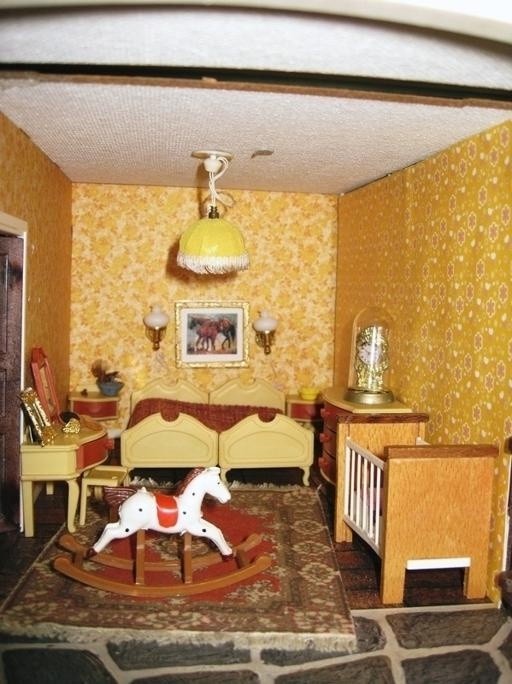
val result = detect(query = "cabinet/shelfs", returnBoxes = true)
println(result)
[316,390,428,491]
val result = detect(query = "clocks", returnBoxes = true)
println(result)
[345,306,394,404]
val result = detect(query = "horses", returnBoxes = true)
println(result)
[189,315,235,352]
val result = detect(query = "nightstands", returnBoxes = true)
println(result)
[285,394,325,423]
[68,391,121,421]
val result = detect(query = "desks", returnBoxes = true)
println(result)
[20,422,114,538]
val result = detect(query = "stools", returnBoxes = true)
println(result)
[79,465,129,526]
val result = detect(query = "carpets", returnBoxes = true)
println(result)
[1,477,359,655]
[0,604,512,684]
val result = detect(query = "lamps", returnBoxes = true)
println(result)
[253,308,278,354]
[143,303,169,350]
[175,149,250,274]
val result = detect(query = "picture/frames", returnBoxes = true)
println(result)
[174,300,249,369]
[22,387,57,446]
[31,346,61,421]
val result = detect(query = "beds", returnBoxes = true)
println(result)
[119,377,219,485]
[208,377,315,488]
[333,421,499,604]
